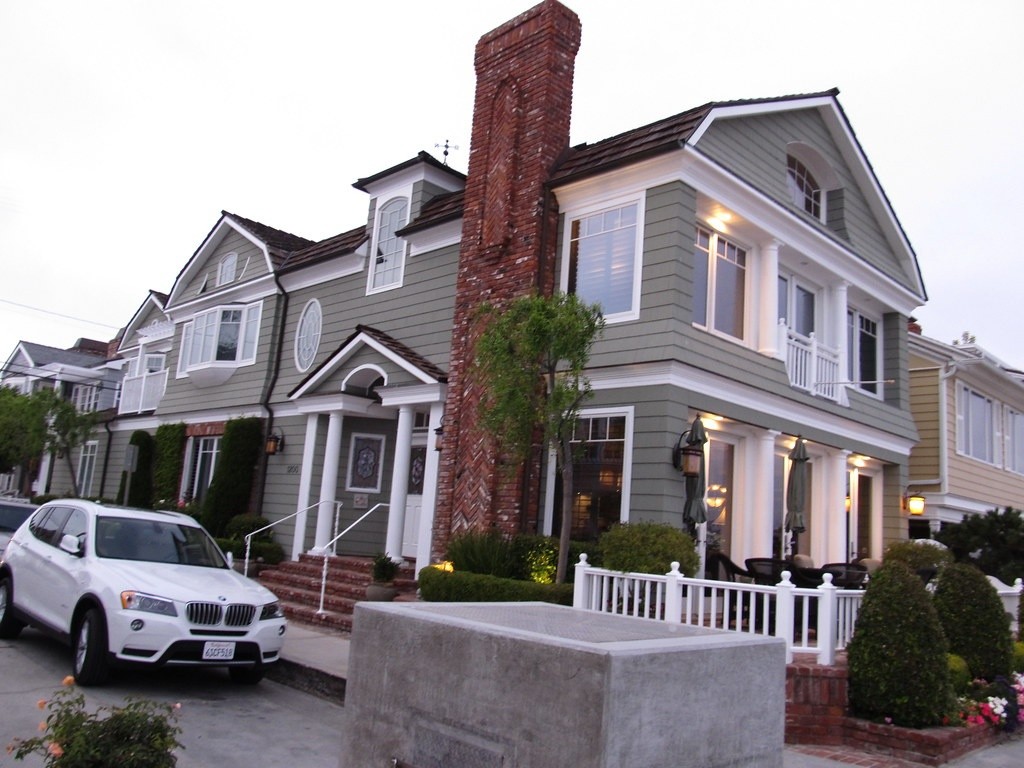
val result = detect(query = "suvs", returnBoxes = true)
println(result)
[0,499,286,688]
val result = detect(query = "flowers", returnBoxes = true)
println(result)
[152,497,192,511]
[7,675,184,767]
[943,669,1023,733]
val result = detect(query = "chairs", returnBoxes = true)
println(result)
[717,551,872,642]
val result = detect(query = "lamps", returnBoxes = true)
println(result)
[902,489,925,515]
[671,429,703,477]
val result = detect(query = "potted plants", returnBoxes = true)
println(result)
[371,552,401,585]
